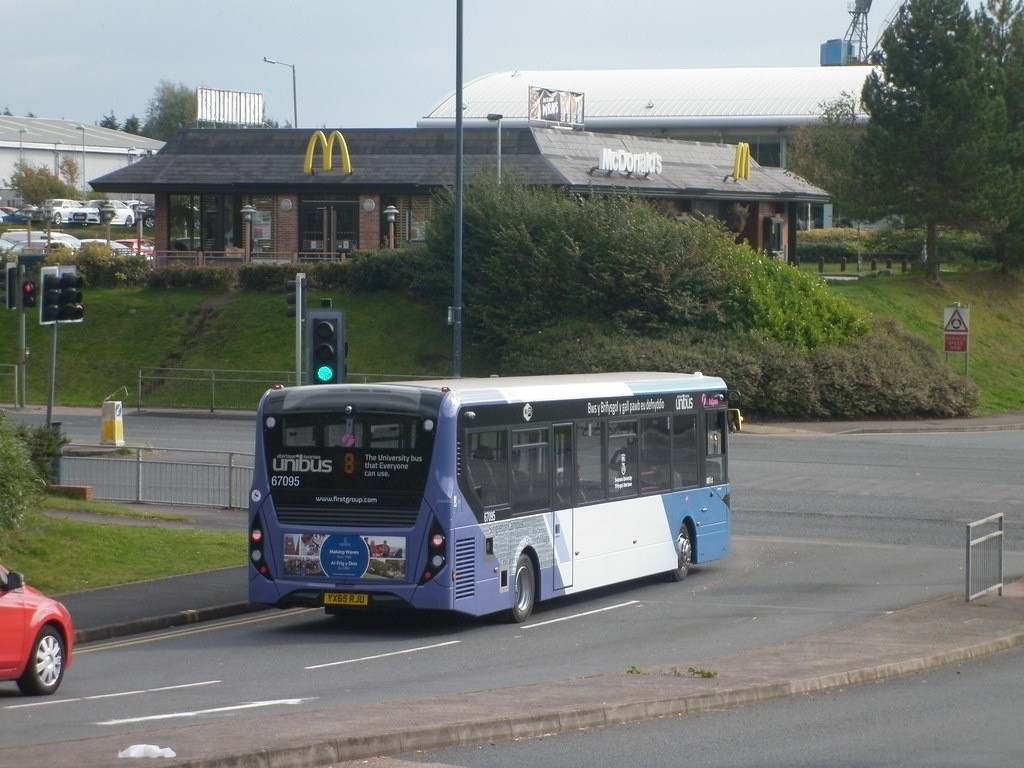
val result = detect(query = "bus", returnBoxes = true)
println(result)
[246,369,743,626]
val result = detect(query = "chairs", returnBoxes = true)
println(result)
[464,456,588,515]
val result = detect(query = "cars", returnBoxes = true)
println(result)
[0,229,157,271]
[0,199,158,227]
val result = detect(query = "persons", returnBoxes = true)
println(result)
[512,450,530,497]
[285,557,319,575]
[364,537,377,557]
[394,548,402,558]
[729,421,736,430]
[300,533,325,556]
[473,446,494,460]
[382,540,390,558]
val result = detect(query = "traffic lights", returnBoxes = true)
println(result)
[24,279,38,308]
[0,260,18,312]
[38,265,62,326]
[57,263,85,326]
[308,311,343,386]
[284,271,307,323]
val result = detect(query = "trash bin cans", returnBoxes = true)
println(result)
[18,254,46,267]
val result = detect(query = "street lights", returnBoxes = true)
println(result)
[485,111,502,195]
[76,124,86,202]
[101,203,116,258]
[20,127,30,208]
[54,138,64,183]
[383,205,399,254]
[44,202,56,256]
[262,55,300,130]
[24,204,38,260]
[239,202,257,264]
